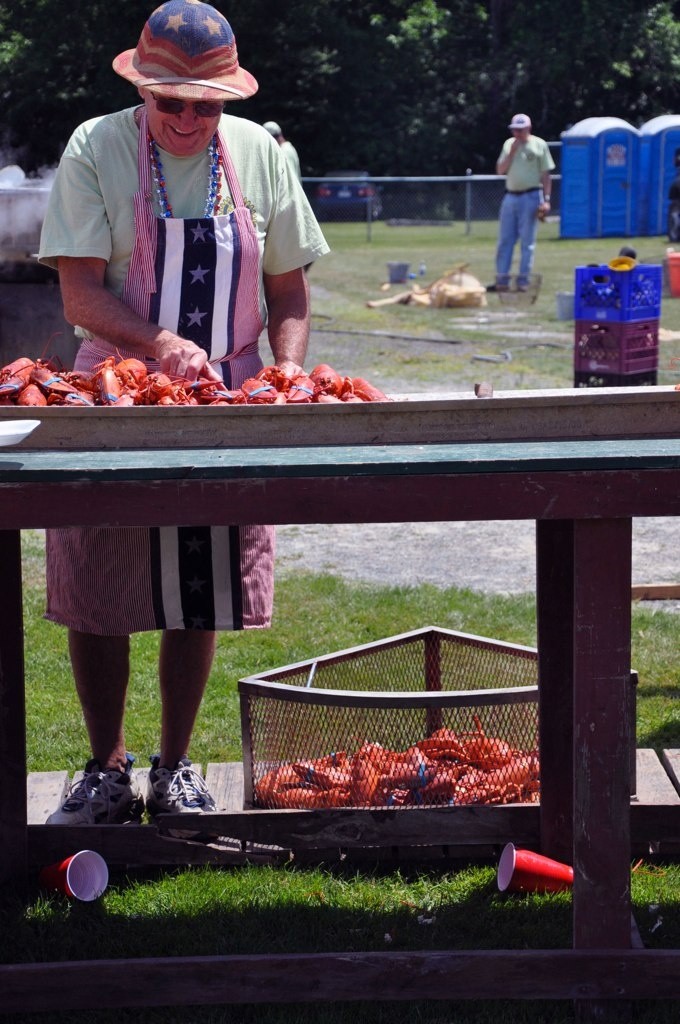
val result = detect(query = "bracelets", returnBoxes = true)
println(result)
[545,195,550,201]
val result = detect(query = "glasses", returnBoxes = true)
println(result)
[151,91,226,118]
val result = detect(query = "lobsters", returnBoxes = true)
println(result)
[0,333,399,405]
[253,715,541,808]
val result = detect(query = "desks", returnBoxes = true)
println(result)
[0,435,680,1008]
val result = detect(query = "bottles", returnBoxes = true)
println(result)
[419,259,425,275]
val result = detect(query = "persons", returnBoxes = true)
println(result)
[486,113,556,293]
[36,1,330,826]
[261,122,301,182]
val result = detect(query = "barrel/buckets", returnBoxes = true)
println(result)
[556,291,575,321]
[388,261,410,284]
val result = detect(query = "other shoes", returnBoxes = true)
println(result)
[517,285,528,291]
[487,284,510,290]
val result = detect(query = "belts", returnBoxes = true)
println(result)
[506,186,539,195]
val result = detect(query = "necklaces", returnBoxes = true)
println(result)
[148,132,223,219]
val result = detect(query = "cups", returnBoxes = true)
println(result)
[497,843,574,893]
[42,849,108,902]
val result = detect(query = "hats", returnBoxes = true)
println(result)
[508,114,531,129]
[112,0,258,102]
[263,121,281,136]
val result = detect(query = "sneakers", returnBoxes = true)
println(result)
[45,754,145,826]
[144,755,220,816]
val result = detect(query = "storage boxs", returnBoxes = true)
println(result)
[573,264,662,387]
[664,251,680,298]
